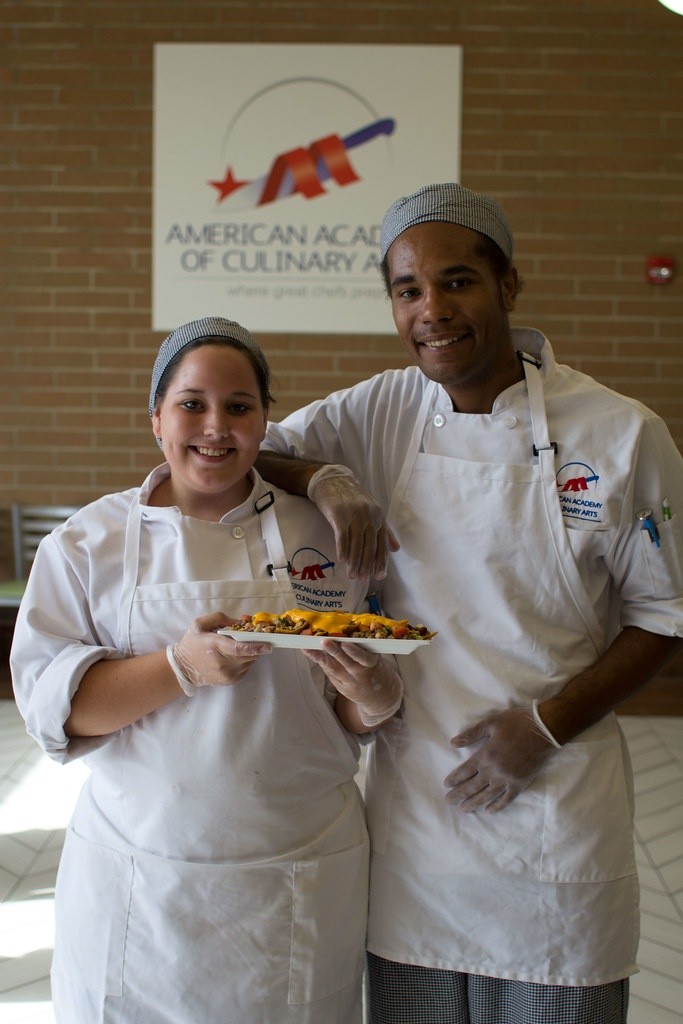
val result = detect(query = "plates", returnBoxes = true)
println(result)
[217,625,438,654]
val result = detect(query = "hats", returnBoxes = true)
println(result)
[147,317,269,452]
[379,183,514,266]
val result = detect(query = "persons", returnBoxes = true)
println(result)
[253,183,683,1024]
[8,317,402,1024]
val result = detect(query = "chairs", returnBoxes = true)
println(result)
[0,503,82,609]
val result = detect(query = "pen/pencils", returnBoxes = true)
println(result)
[636,507,661,549]
[661,496,672,522]
